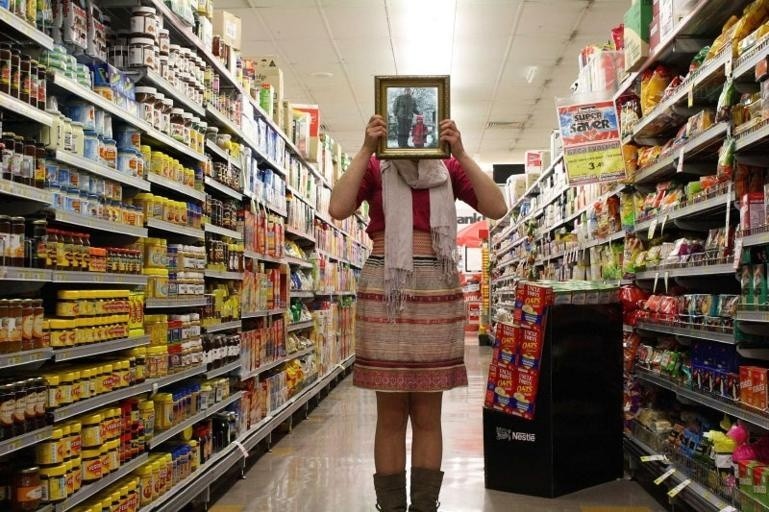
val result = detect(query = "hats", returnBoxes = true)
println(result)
[416,115,424,120]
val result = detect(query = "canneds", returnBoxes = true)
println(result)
[1,6,240,512]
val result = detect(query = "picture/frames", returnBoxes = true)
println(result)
[369,70,453,160]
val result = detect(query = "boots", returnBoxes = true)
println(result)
[408,466,444,512]
[373,468,406,512]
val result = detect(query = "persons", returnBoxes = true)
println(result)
[327,114,509,512]
[393,88,416,147]
[409,114,429,147]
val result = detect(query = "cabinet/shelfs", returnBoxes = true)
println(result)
[1,0,373,512]
[481,0,768,511]
